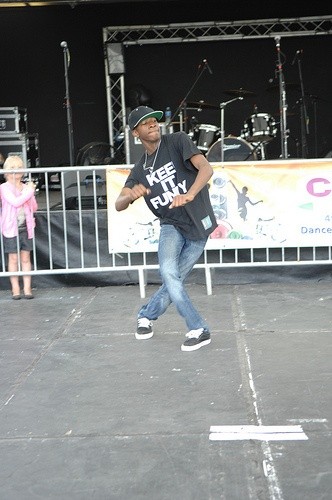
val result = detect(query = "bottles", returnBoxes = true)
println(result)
[165,107,172,124]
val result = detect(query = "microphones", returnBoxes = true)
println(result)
[240,88,242,98]
[275,36,281,49]
[61,41,70,67]
[204,59,212,75]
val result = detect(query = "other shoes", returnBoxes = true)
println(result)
[23,290,33,298]
[12,290,21,299]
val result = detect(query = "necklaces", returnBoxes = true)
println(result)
[143,140,161,175]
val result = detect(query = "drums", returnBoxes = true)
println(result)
[239,123,264,161]
[242,112,278,142]
[206,134,258,162]
[188,132,194,140]
[194,123,221,154]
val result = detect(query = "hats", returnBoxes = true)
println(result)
[128,106,163,131]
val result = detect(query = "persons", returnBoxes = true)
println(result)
[116,106,218,352]
[0,155,38,299]
[228,180,263,220]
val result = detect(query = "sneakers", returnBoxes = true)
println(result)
[181,328,211,351]
[135,315,154,340]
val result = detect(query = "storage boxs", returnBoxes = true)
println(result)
[0,107,39,196]
